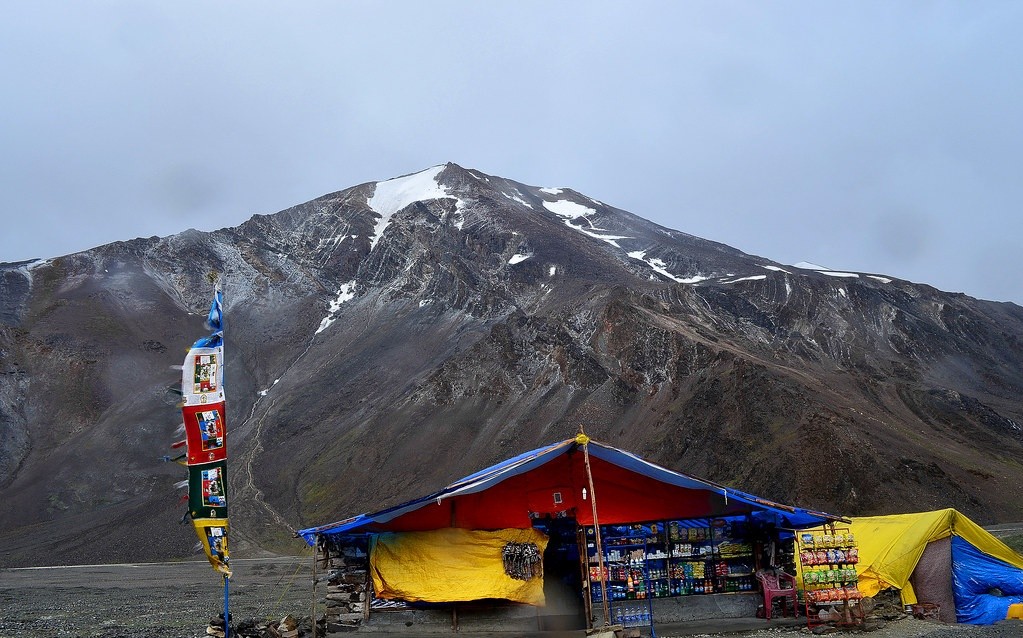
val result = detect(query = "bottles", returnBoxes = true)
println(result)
[586,525,757,627]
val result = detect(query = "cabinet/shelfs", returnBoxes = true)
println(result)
[586,535,759,603]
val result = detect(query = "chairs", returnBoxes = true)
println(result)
[756,568,799,622]
